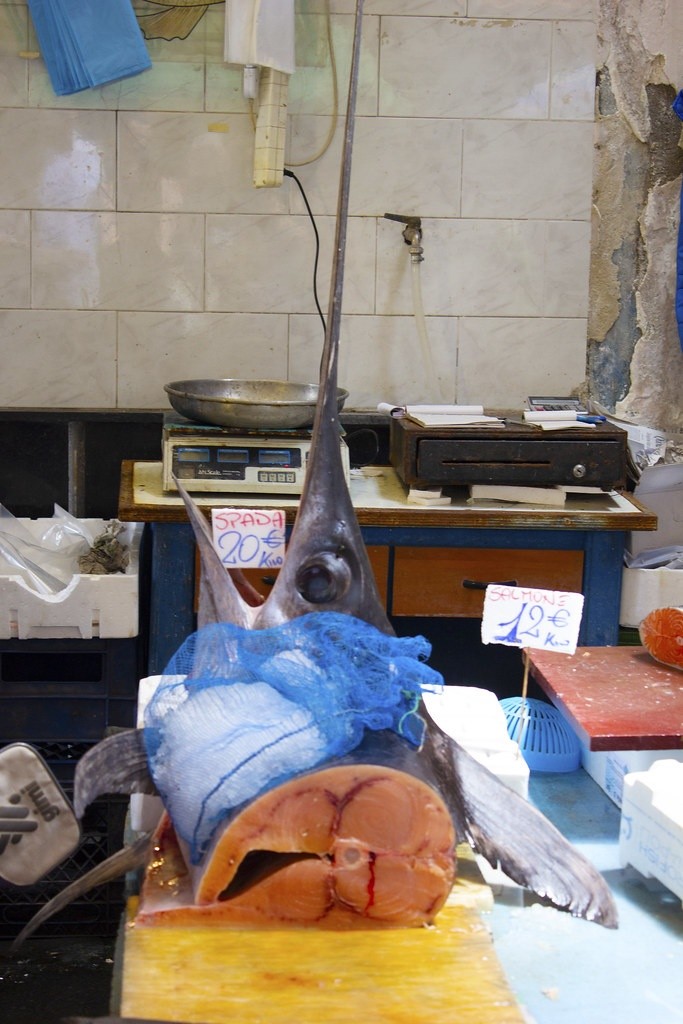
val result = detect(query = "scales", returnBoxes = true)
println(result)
[161,374,352,505]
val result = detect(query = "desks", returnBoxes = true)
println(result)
[118,461,660,677]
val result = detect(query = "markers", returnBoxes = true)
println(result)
[577,415,607,425]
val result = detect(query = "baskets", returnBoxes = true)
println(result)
[500,697,580,772]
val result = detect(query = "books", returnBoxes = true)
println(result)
[376,401,407,419]
[404,404,506,428]
[521,409,597,431]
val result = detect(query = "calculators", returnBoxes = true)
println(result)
[528,395,589,414]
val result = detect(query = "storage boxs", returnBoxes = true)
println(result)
[0,518,147,639]
[619,564,683,627]
[618,758,682,902]
[581,749,683,811]
[624,434,683,558]
